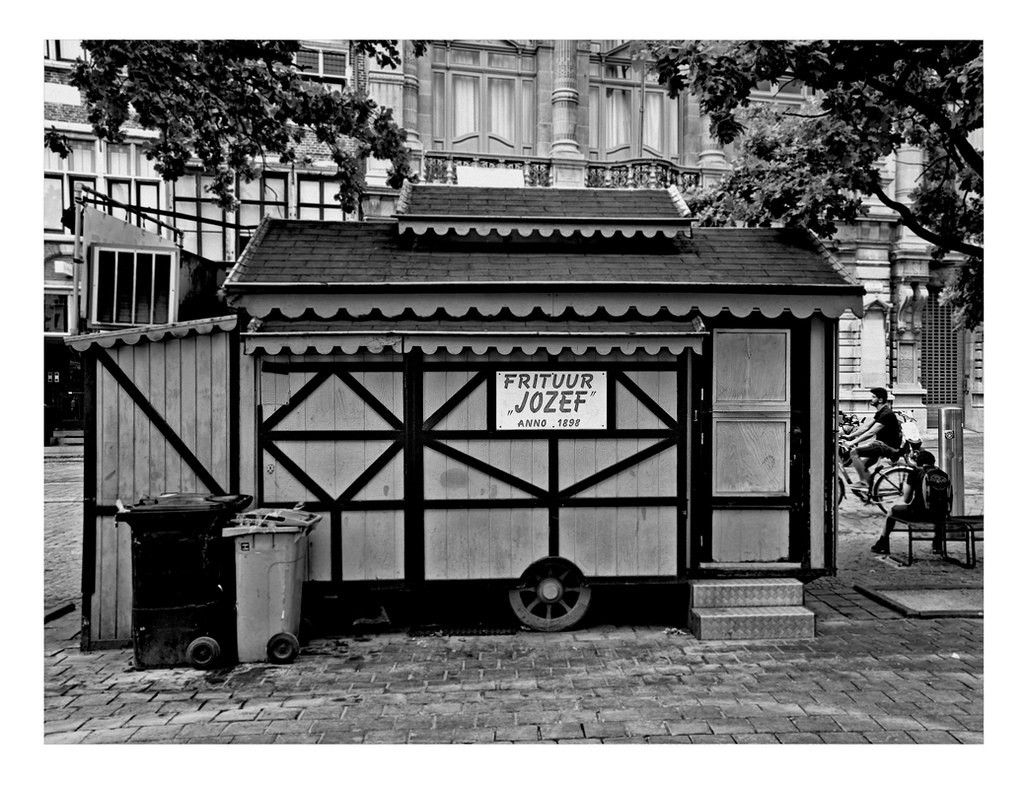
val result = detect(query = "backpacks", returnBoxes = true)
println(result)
[921,464,951,512]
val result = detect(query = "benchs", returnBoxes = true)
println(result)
[885,515,984,569]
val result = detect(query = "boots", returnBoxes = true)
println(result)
[932,536,941,553]
[871,536,890,554]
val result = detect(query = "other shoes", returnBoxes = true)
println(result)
[849,481,869,491]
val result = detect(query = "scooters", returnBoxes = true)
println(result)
[833,410,867,467]
[892,409,926,467]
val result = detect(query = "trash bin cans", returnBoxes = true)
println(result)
[114,491,255,670]
[221,506,323,664]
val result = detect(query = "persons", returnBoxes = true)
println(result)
[870,450,946,553]
[842,388,903,488]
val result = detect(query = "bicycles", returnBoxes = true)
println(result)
[832,440,918,514]
